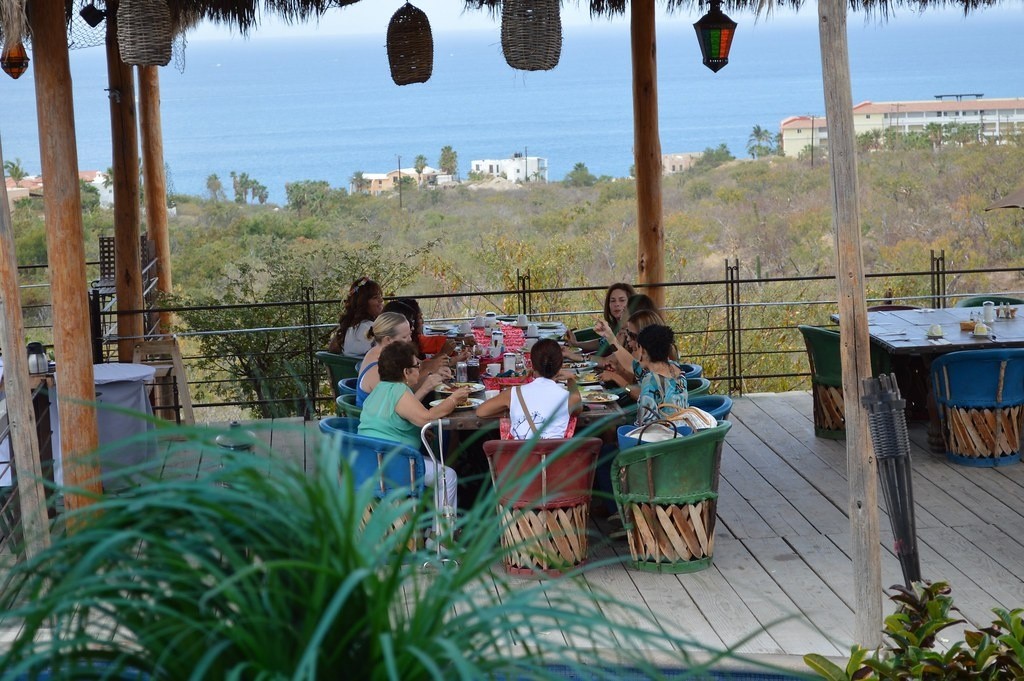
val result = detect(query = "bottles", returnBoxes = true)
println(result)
[484,311,496,327]
[969,301,1012,322]
[503,353,516,373]
[491,331,503,346]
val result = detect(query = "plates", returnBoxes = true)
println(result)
[425,321,619,411]
[924,333,948,338]
[968,331,992,338]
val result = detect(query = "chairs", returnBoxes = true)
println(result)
[86,235,148,360]
[313,326,734,580]
[797,294,1024,467]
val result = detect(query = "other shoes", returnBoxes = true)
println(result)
[425,537,466,555]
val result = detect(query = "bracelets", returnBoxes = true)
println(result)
[447,397,458,409]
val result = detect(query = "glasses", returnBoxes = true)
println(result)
[626,329,639,341]
[410,319,415,329]
[410,361,420,369]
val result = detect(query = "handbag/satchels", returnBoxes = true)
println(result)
[614,406,692,451]
[657,403,718,430]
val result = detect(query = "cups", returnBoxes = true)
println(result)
[457,322,472,333]
[486,363,501,377]
[517,315,528,325]
[488,347,501,359]
[975,323,992,334]
[528,324,538,336]
[485,328,492,336]
[484,390,500,401]
[468,359,480,382]
[928,324,942,335]
[523,339,538,350]
[471,317,484,328]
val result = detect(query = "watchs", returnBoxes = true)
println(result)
[624,384,632,393]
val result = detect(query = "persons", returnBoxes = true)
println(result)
[329,277,473,496]
[562,283,696,444]
[357,341,471,556]
[588,315,689,538]
[476,339,583,440]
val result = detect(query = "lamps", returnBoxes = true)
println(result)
[217,420,258,492]
[692,0,738,73]
[0,19,30,79]
[80,4,109,28]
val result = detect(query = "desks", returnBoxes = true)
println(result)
[830,304,1024,428]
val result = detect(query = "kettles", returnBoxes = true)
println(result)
[26,342,48,374]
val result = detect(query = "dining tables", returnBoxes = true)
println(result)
[422,321,626,536]
